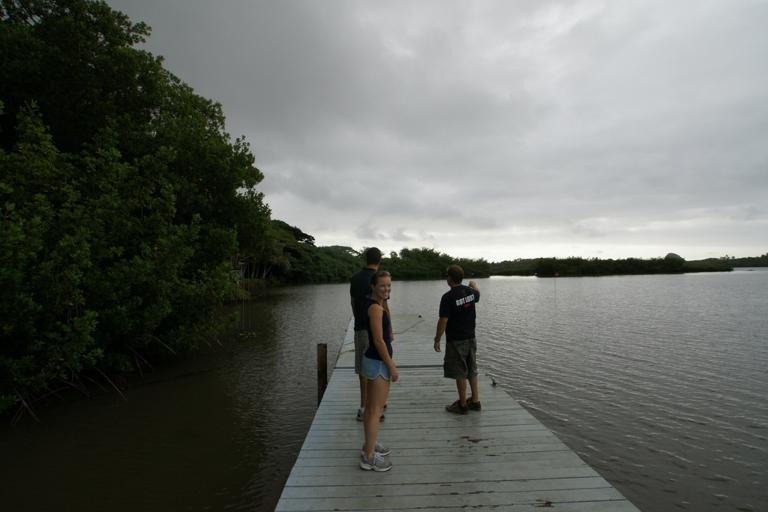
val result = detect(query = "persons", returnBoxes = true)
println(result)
[432,263,481,415]
[357,269,399,472]
[348,247,389,424]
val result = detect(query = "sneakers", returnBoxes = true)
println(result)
[446,396,481,415]
[359,439,393,471]
[354,402,388,423]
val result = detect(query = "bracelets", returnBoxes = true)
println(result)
[434,336,441,342]
